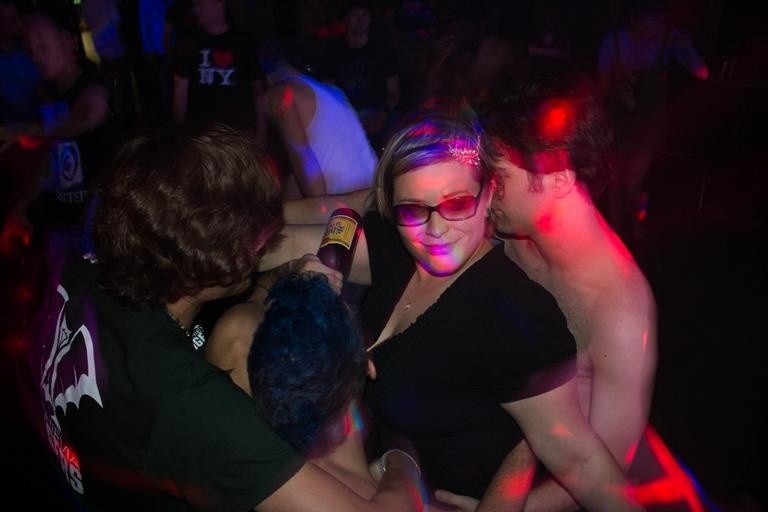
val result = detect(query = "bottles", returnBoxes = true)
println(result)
[317,206,363,283]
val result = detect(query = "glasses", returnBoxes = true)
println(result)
[393,184,483,227]
